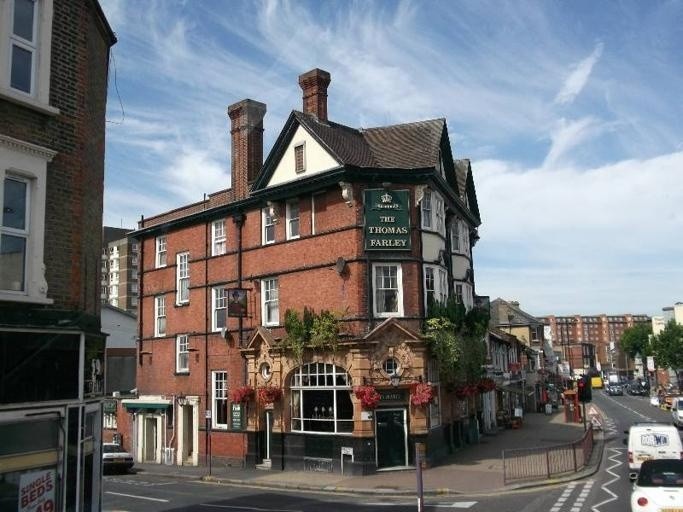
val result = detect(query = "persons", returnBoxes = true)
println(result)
[227,291,247,317]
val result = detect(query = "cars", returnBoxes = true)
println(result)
[603,377,683,512]
[102,442,133,474]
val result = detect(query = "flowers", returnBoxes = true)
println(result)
[229,384,283,408]
[351,384,380,407]
[410,382,433,407]
[457,377,496,398]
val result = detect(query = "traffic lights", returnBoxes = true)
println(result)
[577,379,586,402]
